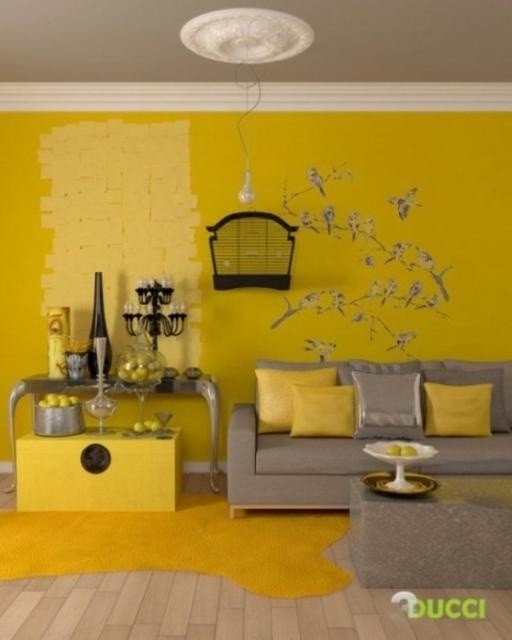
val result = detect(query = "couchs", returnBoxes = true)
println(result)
[227,358,510,515]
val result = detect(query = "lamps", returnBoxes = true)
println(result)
[125,278,184,350]
[177,10,315,204]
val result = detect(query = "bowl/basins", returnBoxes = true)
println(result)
[33,404,85,437]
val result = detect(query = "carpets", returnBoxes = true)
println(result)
[0,490,356,601]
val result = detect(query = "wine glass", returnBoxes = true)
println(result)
[119,378,160,435]
[362,439,439,491]
[84,402,117,436]
[151,408,173,437]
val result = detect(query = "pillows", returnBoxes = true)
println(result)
[353,368,419,440]
[253,365,340,436]
[289,383,357,439]
[425,383,491,436]
[420,368,510,433]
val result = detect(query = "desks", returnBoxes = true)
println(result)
[7,377,225,497]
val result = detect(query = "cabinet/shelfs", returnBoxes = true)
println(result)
[13,426,182,515]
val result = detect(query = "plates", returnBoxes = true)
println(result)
[360,471,440,497]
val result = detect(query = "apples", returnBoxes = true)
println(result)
[400,446,417,457]
[39,394,80,407]
[387,444,400,456]
[118,351,166,383]
[133,420,161,433]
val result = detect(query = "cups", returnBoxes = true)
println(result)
[65,350,88,378]
